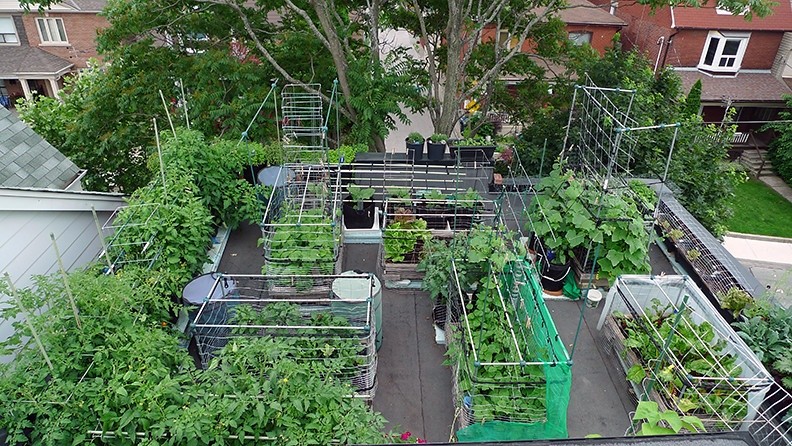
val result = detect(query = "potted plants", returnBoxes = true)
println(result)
[420,240,477,333]
[342,183,376,230]
[527,173,578,257]
[467,230,519,313]
[450,188,484,224]
[405,132,426,159]
[448,137,496,162]
[382,188,412,219]
[614,304,747,418]
[541,201,595,292]
[425,133,447,161]
[415,188,452,229]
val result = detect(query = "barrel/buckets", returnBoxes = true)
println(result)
[182,272,241,364]
[332,270,383,353]
[258,166,296,234]
